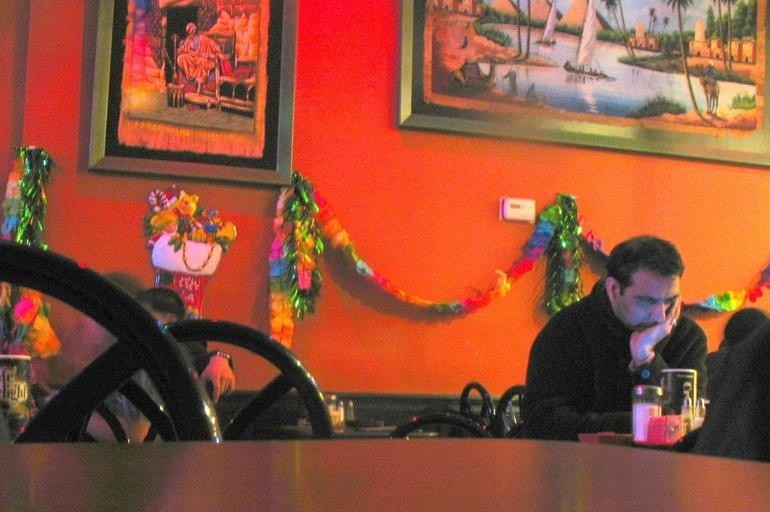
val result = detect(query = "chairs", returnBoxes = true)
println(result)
[83,319,334,441]
[385,381,525,440]
[0,240,221,441]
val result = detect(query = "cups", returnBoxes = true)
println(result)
[363,416,386,433]
[660,367,697,414]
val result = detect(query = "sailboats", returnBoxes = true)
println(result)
[534,2,558,45]
[563,0,615,77]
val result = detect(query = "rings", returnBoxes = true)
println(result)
[672,320,677,328]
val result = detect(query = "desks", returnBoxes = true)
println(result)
[0,438,769,512]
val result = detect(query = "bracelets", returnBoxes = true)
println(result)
[211,349,231,362]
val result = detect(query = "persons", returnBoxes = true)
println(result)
[722,308,768,352]
[178,23,221,92]
[521,233,710,437]
[681,316,769,461]
[137,287,237,401]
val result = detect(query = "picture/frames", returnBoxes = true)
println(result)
[88,0,297,186]
[397,0,770,167]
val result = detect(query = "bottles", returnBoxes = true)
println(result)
[326,394,355,432]
[681,397,706,438]
[631,364,664,441]
